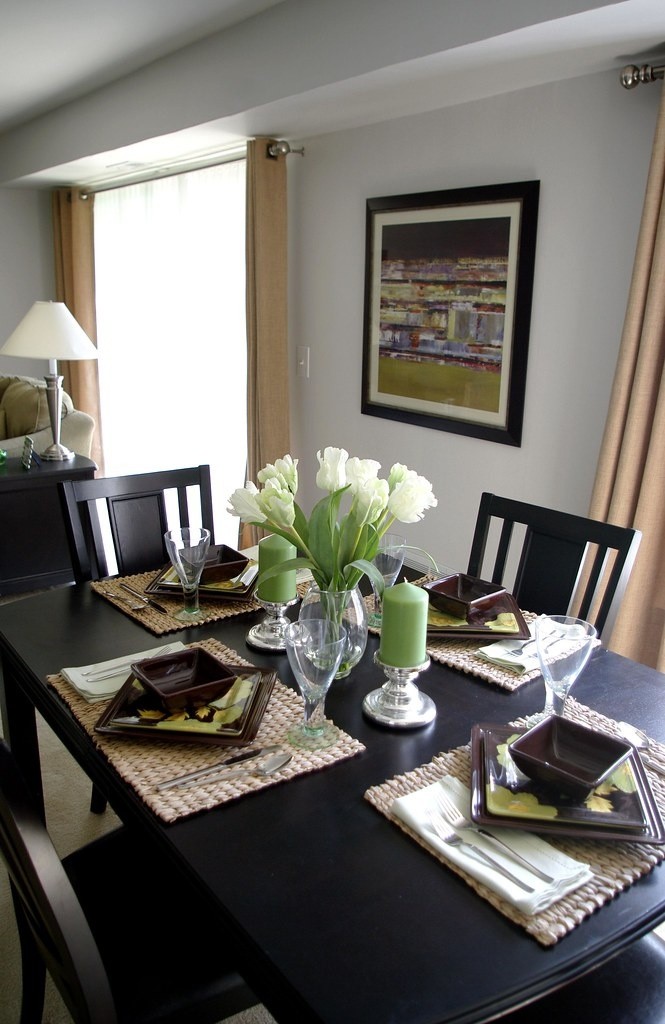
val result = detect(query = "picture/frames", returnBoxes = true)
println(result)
[361,179,541,448]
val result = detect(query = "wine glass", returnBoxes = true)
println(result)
[284,618,348,750]
[525,615,598,729]
[164,528,211,622]
[366,532,406,627]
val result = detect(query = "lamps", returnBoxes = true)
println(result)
[0,299,100,462]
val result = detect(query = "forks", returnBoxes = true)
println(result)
[427,807,535,894]
[86,645,173,683]
[103,591,151,611]
[436,794,554,883]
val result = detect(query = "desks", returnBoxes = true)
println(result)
[0,550,665,1024]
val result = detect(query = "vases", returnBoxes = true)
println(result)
[299,586,367,680]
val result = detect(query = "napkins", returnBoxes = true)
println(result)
[391,775,597,918]
[238,544,315,587]
[60,641,187,705]
[473,612,587,676]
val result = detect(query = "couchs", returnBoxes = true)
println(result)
[0,374,94,459]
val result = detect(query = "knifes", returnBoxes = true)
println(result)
[157,745,283,792]
[119,583,168,615]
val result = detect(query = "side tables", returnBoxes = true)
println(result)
[0,454,96,601]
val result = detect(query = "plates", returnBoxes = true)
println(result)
[427,592,532,641]
[93,657,278,746]
[470,723,665,845]
[144,559,259,602]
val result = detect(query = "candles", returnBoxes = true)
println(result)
[256,534,296,602]
[381,577,428,669]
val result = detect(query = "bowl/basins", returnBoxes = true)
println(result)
[130,646,239,713]
[178,544,249,585]
[508,715,633,804]
[420,573,507,622]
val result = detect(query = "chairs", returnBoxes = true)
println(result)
[466,492,642,648]
[59,464,214,813]
[0,733,261,1024]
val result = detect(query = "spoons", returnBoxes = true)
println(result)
[177,752,293,790]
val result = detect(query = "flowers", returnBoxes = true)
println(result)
[226,447,437,596]
